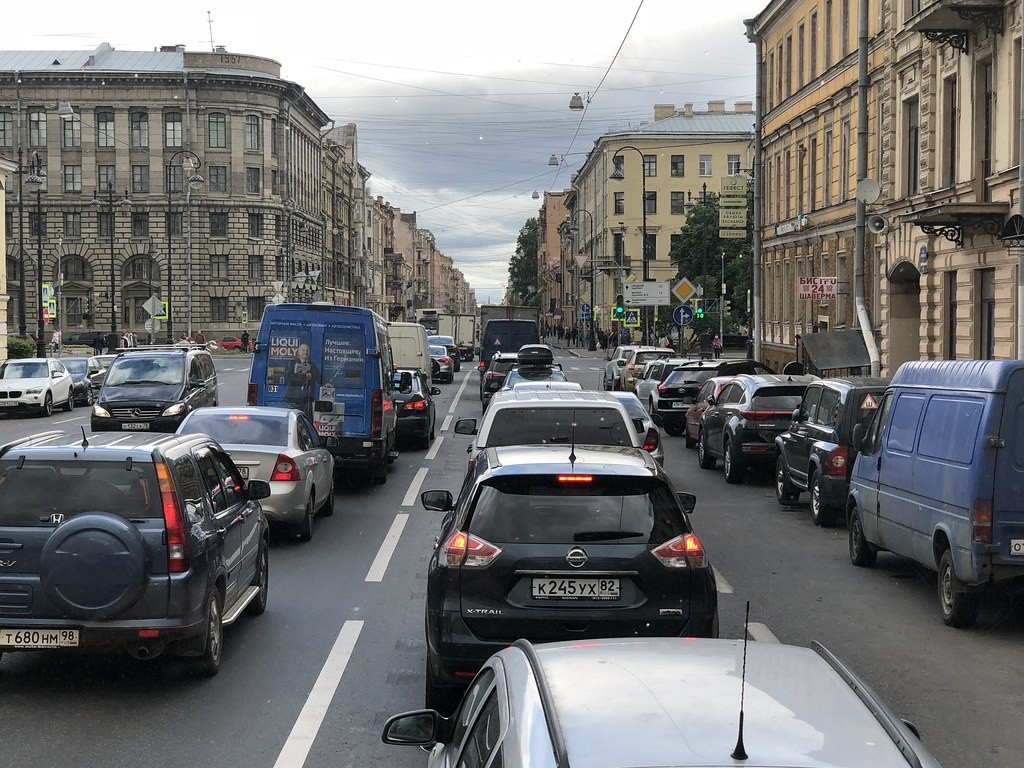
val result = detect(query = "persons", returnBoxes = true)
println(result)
[284,343,325,425]
[544,321,556,338]
[92,332,106,356]
[658,332,674,348]
[241,330,250,353]
[597,328,618,351]
[179,332,190,344]
[712,336,721,359]
[558,323,590,347]
[51,328,60,353]
[195,330,205,344]
[120,332,138,348]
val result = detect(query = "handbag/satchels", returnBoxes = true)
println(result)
[665,338,668,346]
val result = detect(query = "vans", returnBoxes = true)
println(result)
[386,322,434,390]
[845,358,1024,629]
[473,319,540,384]
[248,303,402,485]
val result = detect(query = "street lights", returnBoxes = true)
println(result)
[721,252,725,356]
[166,149,207,342]
[610,145,650,343]
[285,211,310,302]
[562,209,598,351]
[11,147,48,341]
[27,150,49,360]
[87,179,133,356]
[686,182,720,358]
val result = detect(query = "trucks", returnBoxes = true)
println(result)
[438,314,479,361]
[479,304,541,337]
[416,308,449,335]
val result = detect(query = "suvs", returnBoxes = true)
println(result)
[620,347,677,391]
[697,373,824,485]
[657,356,777,437]
[503,343,568,388]
[771,378,891,527]
[600,345,655,391]
[89,344,219,433]
[477,349,519,413]
[0,433,273,677]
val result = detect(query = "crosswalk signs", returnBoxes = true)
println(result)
[611,307,625,322]
[624,309,641,328]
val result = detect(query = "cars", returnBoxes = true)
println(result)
[176,405,336,543]
[513,381,582,392]
[426,335,462,384]
[63,331,109,349]
[391,369,442,446]
[58,353,122,410]
[417,442,721,688]
[452,390,643,454]
[682,377,739,448]
[381,639,943,768]
[0,359,74,419]
[634,358,705,424]
[610,391,664,466]
[214,336,254,352]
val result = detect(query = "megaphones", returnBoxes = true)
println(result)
[868,215,888,234]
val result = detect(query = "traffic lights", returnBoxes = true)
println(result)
[615,294,625,319]
[696,313,704,321]
[724,300,732,318]
[689,298,698,315]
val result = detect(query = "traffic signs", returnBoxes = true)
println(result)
[620,283,671,306]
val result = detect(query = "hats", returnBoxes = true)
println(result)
[715,336,718,338]
